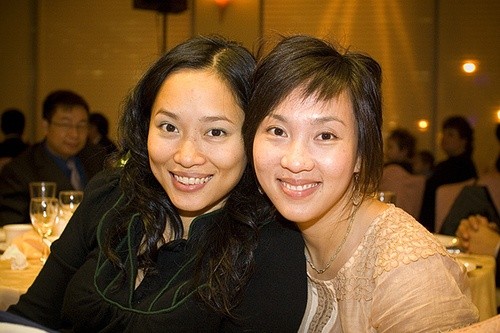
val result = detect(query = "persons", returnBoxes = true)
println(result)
[245,35,479,333]
[0,88,120,225]
[382,126,436,178]
[7,33,308,333]
[417,114,480,235]
[455,212,500,291]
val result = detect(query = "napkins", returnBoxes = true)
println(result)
[0,244,28,272]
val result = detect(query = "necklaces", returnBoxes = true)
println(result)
[304,192,361,275]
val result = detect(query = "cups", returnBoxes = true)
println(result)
[5,225,33,248]
[59,192,82,221]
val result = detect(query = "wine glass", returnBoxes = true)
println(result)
[29,183,58,266]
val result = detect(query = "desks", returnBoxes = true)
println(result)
[456,252,497,321]
[0,227,52,313]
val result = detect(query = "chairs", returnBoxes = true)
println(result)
[357,165,500,333]
